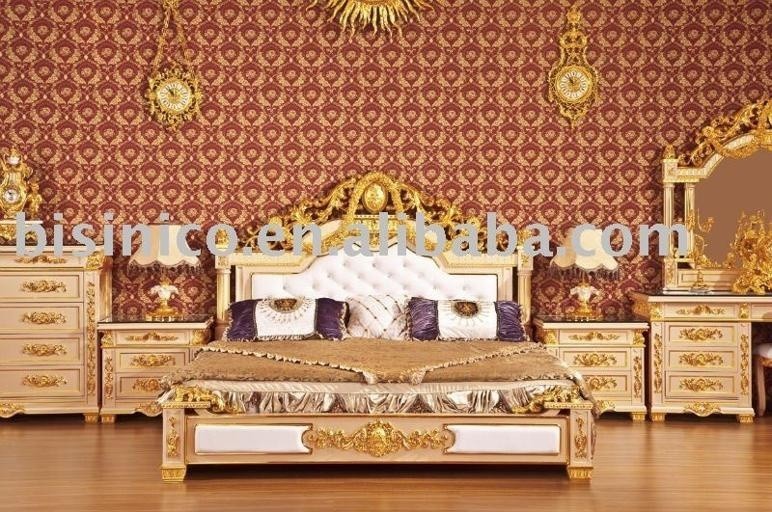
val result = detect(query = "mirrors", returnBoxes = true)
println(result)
[655,99,772,295]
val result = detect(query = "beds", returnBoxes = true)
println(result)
[157,168,599,484]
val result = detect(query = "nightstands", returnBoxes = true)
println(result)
[96,312,215,427]
[534,307,651,426]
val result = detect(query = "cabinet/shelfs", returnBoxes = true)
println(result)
[0,243,113,424]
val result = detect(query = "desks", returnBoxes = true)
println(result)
[632,288,771,423]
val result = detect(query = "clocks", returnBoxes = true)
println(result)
[142,1,204,126]
[546,6,601,129]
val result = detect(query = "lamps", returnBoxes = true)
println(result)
[548,227,624,322]
[128,212,202,321]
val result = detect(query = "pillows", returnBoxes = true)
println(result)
[218,294,530,344]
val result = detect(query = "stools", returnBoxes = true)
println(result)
[750,340,771,417]
